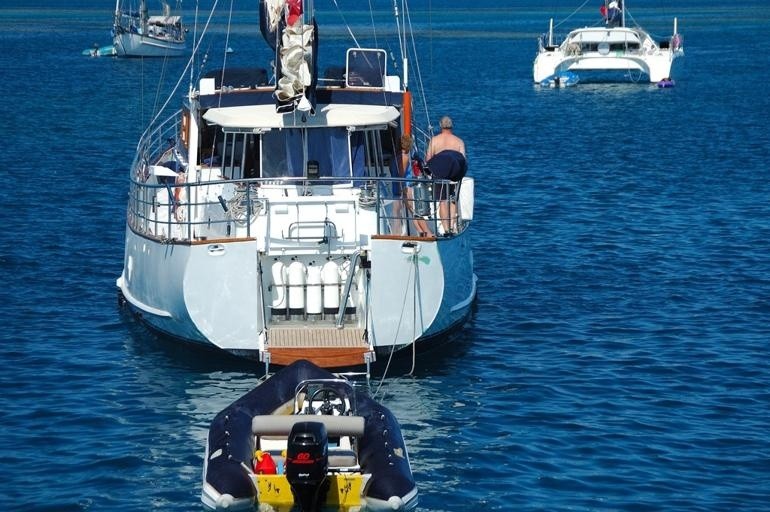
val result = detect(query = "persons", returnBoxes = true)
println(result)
[388,135,436,239]
[424,116,467,236]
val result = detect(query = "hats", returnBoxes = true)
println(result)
[440,117,453,128]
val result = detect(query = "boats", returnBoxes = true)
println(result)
[198,358,419,512]
[115,1,479,370]
[530,1,684,88]
[81,0,187,59]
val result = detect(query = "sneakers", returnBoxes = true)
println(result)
[446,228,454,234]
